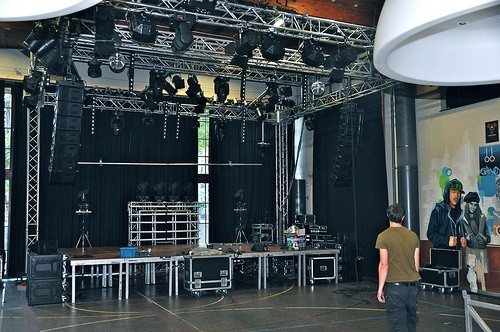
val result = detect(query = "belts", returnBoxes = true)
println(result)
[385,282,415,286]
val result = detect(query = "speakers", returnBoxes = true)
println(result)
[333,97,365,187]
[27,252,63,307]
[50,82,85,183]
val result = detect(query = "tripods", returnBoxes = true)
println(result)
[75,215,93,255]
[233,212,249,244]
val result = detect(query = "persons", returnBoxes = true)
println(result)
[485,121,499,143]
[376,204,420,332]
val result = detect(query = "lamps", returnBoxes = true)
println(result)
[234,189,244,199]
[20,0,358,141]
[77,188,89,203]
[137,182,194,202]
[256,148,267,158]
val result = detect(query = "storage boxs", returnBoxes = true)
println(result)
[252,224,274,243]
[417,248,463,288]
[183,254,233,292]
[294,214,334,249]
[120,247,139,258]
[308,257,337,281]
